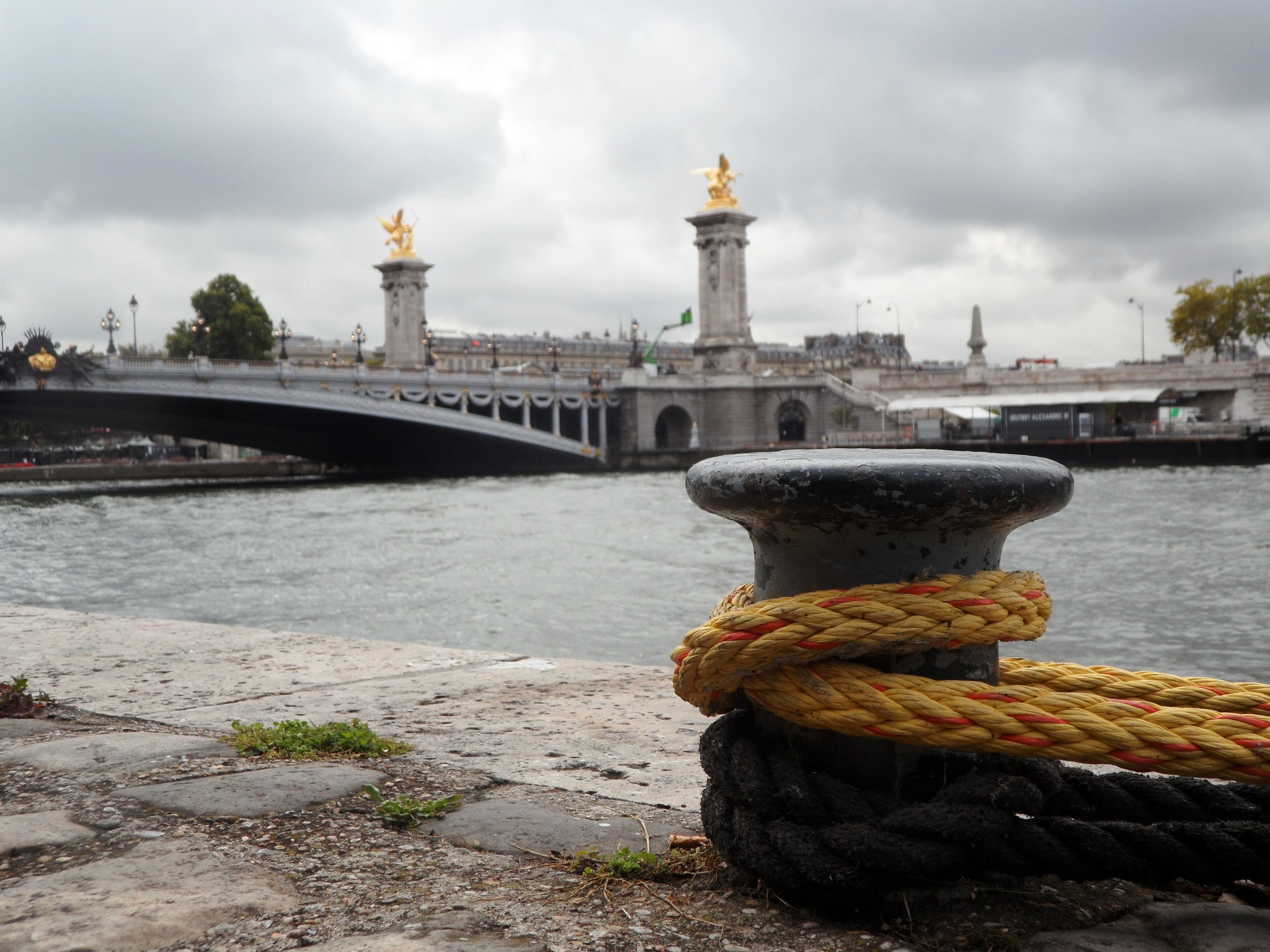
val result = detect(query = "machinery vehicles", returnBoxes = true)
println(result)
[641,307,694,375]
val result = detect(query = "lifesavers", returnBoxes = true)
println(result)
[1151,420,1162,431]
[905,427,913,438]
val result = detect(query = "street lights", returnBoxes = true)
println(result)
[351,322,367,363]
[420,316,429,365]
[1232,268,1244,362]
[630,318,642,367]
[0,316,7,351]
[129,295,139,357]
[192,314,210,357]
[549,336,561,373]
[855,297,871,351]
[1128,296,1146,364]
[101,307,120,354]
[274,318,292,361]
[487,334,502,369]
[886,306,901,371]
